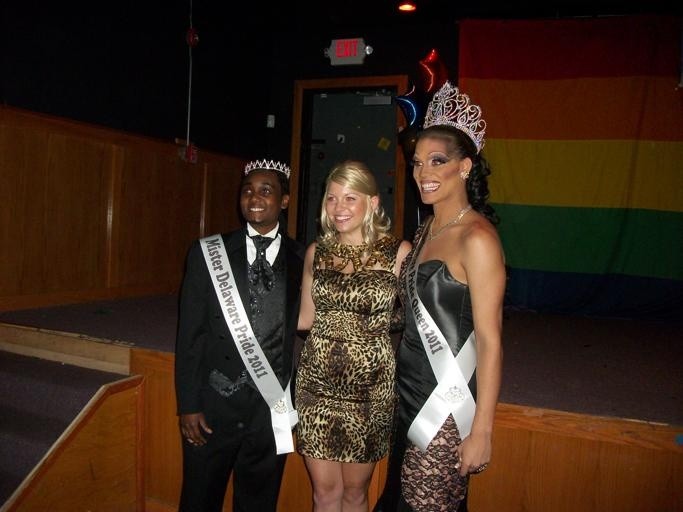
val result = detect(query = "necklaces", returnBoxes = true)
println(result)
[425,203,472,241]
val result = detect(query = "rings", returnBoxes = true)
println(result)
[477,467,485,473]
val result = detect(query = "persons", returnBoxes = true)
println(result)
[174,158,306,512]
[295,160,413,512]
[370,79,507,512]
